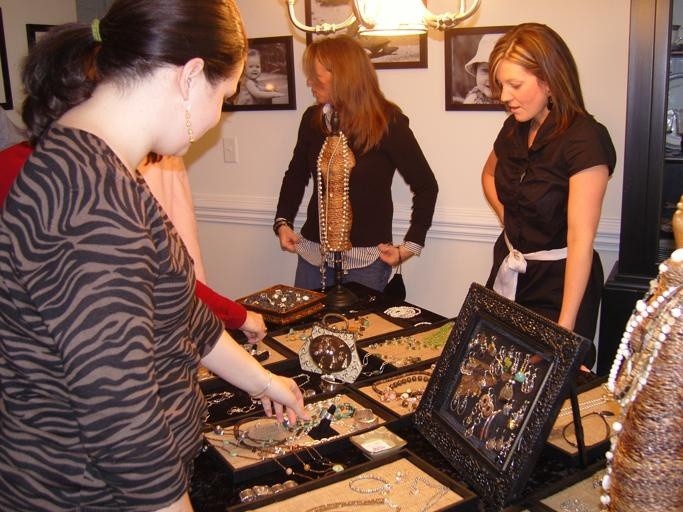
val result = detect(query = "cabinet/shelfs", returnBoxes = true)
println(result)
[618,0,683,282]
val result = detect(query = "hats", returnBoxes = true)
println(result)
[464,33,506,78]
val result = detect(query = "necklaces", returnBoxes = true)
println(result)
[562,408,614,448]
[316,111,348,252]
[556,397,607,417]
[606,286,680,406]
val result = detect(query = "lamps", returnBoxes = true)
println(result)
[286,0,479,38]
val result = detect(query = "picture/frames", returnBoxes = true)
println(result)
[0,7,14,111]
[415,282,592,512]
[221,36,297,111]
[25,23,58,54]
[305,0,428,68]
[445,25,516,111]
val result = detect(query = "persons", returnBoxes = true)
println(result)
[461,33,506,105]
[272,33,438,293]
[602,195,683,511]
[482,22,615,373]
[0,0,307,512]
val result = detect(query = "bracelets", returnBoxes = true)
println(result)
[273,219,290,235]
[348,474,391,493]
[321,312,349,331]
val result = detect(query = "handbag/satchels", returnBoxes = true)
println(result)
[383,273,406,299]
